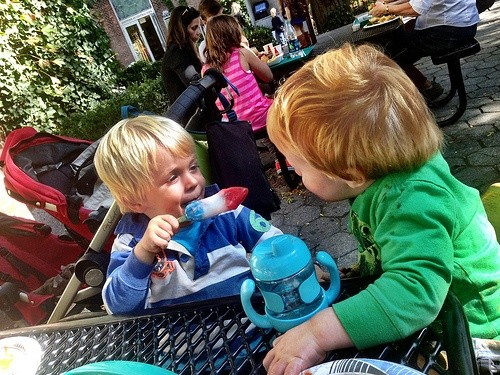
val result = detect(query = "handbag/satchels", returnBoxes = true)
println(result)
[202,89,281,217]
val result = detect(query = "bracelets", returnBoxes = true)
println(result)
[386,5,390,15]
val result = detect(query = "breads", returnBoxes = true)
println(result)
[369,15,395,23]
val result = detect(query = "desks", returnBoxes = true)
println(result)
[0,272,477,375]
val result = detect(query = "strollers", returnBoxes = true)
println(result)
[0,67,224,326]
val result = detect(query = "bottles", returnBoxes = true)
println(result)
[284,20,303,59]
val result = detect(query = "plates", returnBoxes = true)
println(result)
[1,337,41,375]
[361,15,400,29]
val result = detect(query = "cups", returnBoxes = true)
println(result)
[262,44,284,55]
[239,235,342,332]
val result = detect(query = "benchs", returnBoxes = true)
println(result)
[249,123,298,190]
[415,36,481,126]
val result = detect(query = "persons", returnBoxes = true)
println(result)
[94,114,284,375]
[200,14,298,177]
[370,0,480,104]
[160,6,209,133]
[198,0,249,64]
[281,0,310,42]
[267,7,284,46]
[262,44,500,374]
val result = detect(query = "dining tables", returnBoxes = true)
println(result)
[257,13,404,86]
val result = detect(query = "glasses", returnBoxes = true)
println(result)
[181,6,195,16]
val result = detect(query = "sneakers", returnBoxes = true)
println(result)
[421,76,443,103]
[274,160,295,175]
